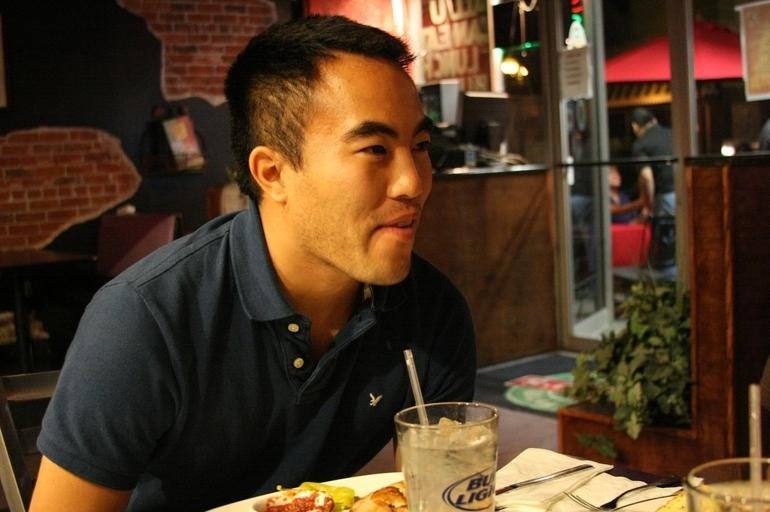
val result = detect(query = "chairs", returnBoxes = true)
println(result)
[611,224,652,283]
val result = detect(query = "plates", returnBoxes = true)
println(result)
[252,472,407,512]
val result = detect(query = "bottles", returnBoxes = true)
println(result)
[464,141,477,167]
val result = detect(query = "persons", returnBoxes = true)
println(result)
[630,108,676,273]
[27,13,476,512]
[607,167,649,229]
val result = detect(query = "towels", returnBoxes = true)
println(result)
[548,473,682,512]
[494,448,614,512]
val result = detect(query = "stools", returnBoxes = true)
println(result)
[0,370,64,512]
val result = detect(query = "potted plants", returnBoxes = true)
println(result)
[556,262,695,486]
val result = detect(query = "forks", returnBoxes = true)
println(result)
[564,482,665,512]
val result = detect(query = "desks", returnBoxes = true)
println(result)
[415,156,557,370]
[1,248,98,376]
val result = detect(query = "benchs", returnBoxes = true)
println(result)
[89,213,184,284]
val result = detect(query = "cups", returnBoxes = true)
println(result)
[685,458,770,512]
[394,402,499,512]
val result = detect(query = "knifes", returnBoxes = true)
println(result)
[495,464,593,496]
